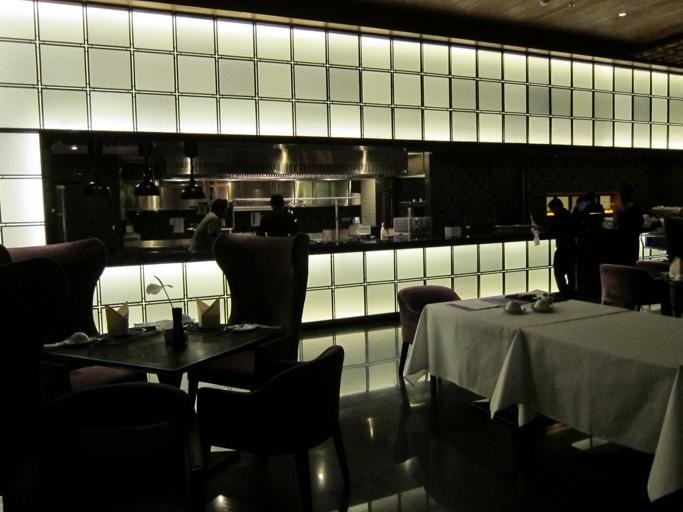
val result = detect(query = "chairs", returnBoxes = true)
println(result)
[196,345,347,510]
[398,283,460,402]
[597,260,673,315]
[4,245,191,505]
[188,236,312,406]
[9,237,149,388]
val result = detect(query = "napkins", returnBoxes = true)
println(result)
[196,298,222,326]
[105,303,130,334]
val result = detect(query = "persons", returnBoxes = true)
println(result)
[534,189,683,296]
[255,193,308,237]
[187,199,229,254]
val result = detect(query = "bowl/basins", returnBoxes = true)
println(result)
[505,301,521,312]
[70,332,89,342]
[534,300,549,310]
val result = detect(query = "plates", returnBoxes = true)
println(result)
[184,325,229,335]
[232,324,257,332]
[64,338,94,346]
[499,306,526,313]
[530,304,553,312]
[96,327,147,342]
